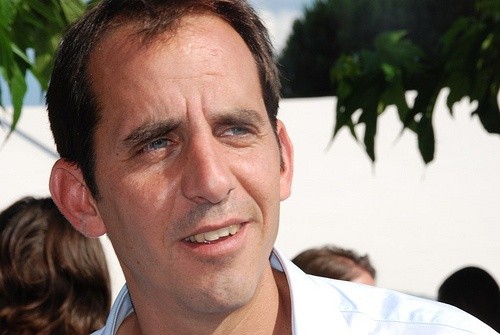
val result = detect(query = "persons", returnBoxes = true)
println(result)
[0,194,112,335]
[435,265,500,333]
[287,245,376,288]
[46,0,500,335]
